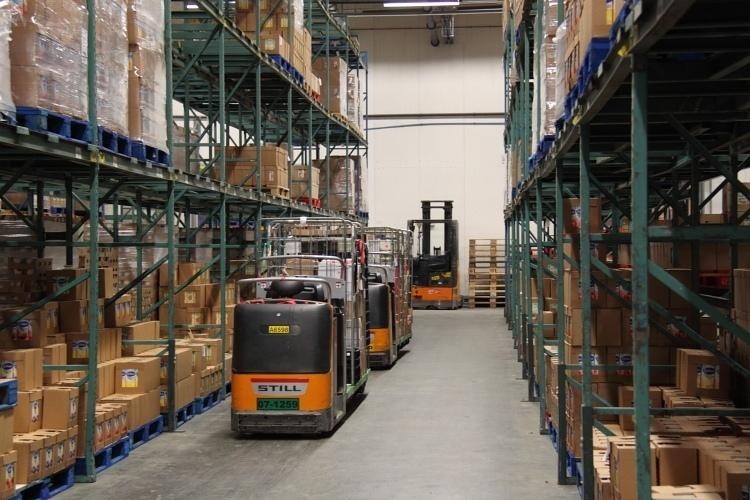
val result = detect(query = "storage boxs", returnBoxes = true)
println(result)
[258,217,414,381]
[314,57,364,130]
[6,2,170,149]
[171,126,200,176]
[517,198,750,498]
[191,0,322,99]
[500,0,631,202]
[0,189,256,499]
[212,143,368,214]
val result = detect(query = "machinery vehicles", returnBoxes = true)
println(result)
[368,227,414,370]
[231,216,371,437]
[406,201,464,310]
